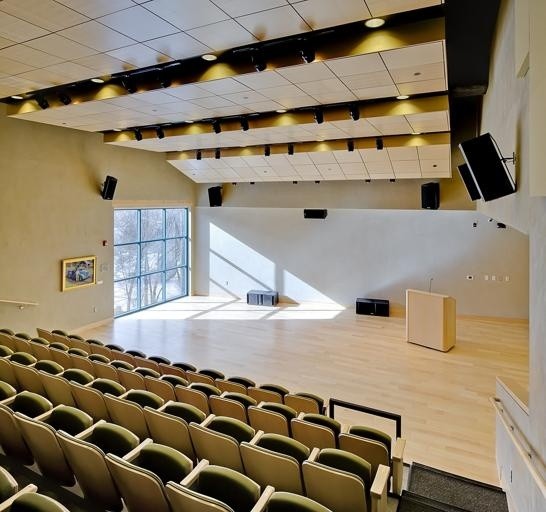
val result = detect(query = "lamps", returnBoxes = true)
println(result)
[132,112,385,161]
[33,40,317,111]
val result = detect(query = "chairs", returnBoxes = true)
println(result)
[0,328,408,511]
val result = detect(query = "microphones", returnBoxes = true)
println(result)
[429,278,433,292]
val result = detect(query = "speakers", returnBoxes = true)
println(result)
[457,164,481,201]
[102,176,117,200]
[458,132,516,202]
[421,183,439,209]
[208,186,223,207]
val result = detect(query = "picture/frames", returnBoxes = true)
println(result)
[61,255,96,291]
